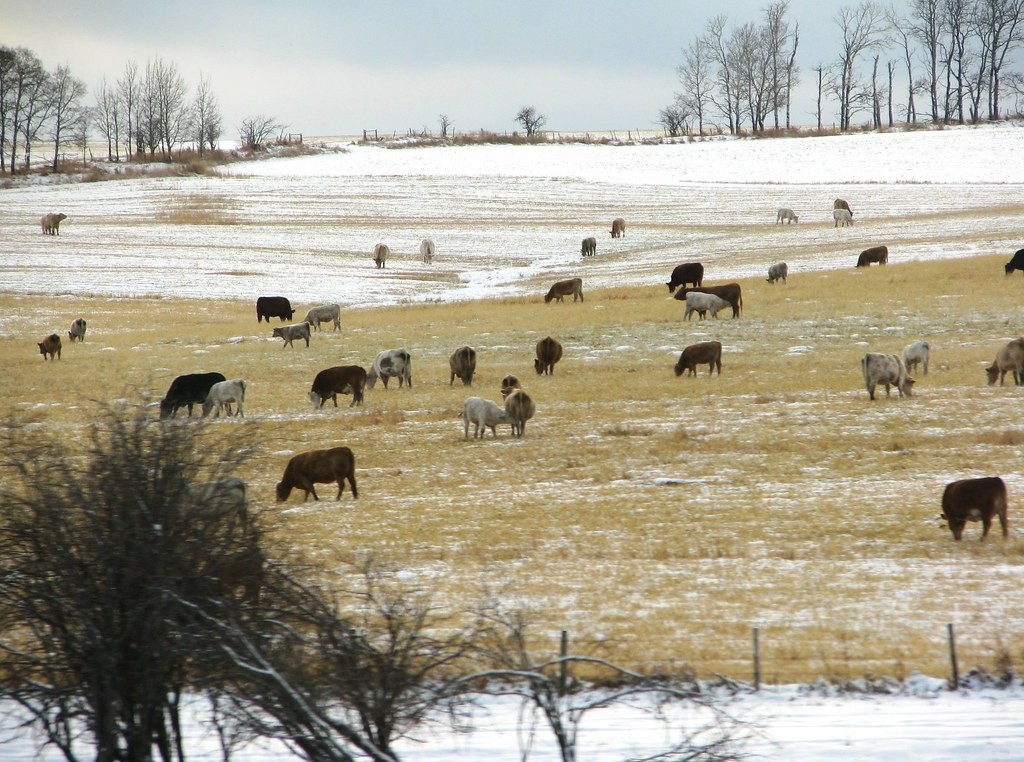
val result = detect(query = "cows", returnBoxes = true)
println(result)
[833,199,854,228]
[274,446,359,504]
[544,278,585,303]
[363,348,413,390]
[939,477,1010,543]
[533,336,563,376]
[256,295,295,324]
[1004,249,1024,276]
[448,346,476,387]
[158,371,247,421]
[853,246,889,267]
[610,217,627,239]
[985,335,1024,388]
[666,262,745,322]
[673,340,722,378]
[307,364,367,410]
[463,375,536,440]
[372,243,390,268]
[860,340,931,400]
[40,213,67,236]
[420,238,437,266]
[38,318,86,362]
[765,260,789,285]
[271,303,342,350]
[580,236,598,257]
[775,208,799,225]
